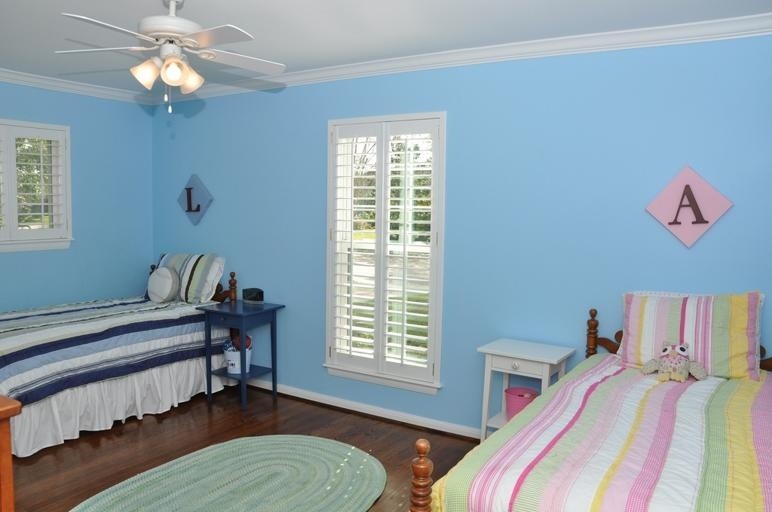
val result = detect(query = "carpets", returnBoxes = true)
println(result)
[67,432,389,510]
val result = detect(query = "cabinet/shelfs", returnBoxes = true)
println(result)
[472,337,576,448]
[193,297,286,413]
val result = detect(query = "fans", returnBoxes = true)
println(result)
[42,0,293,80]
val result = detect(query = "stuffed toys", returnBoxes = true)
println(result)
[640,341,708,385]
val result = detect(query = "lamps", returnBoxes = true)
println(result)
[123,48,207,116]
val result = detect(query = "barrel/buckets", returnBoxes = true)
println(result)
[224,348,252,378]
[504,387,538,422]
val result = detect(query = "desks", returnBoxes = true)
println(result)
[0,390,26,512]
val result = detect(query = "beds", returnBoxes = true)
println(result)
[409,302,770,512]
[0,264,242,465]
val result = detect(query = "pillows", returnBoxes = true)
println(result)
[146,266,181,303]
[615,285,765,383]
[148,249,226,306]
